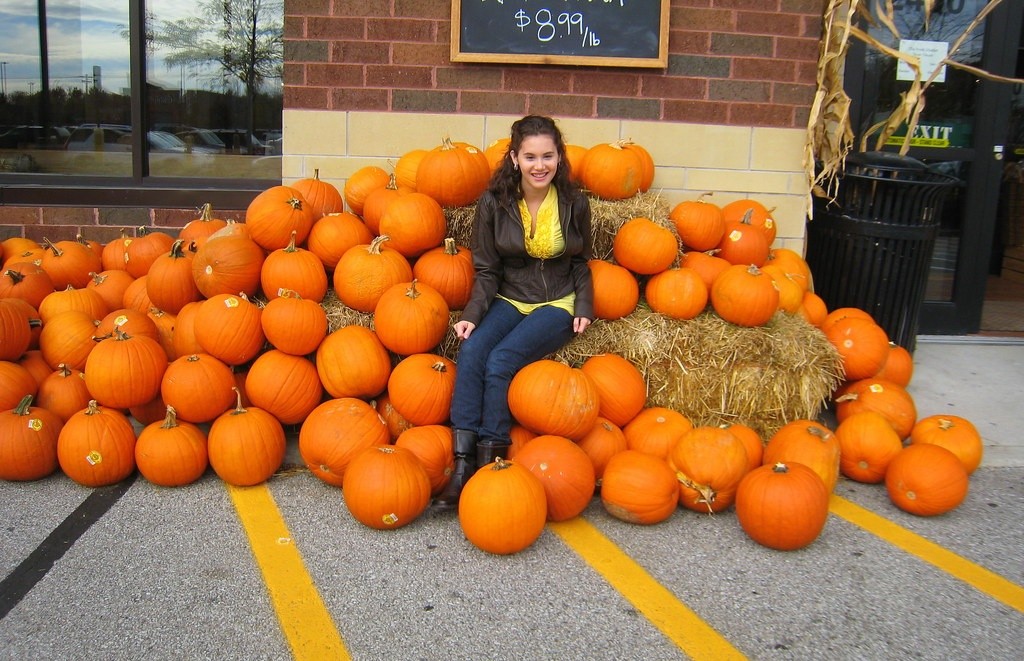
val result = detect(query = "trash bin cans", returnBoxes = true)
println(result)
[804,164,967,356]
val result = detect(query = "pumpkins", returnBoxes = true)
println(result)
[0,135,517,530]
[459,138,982,551]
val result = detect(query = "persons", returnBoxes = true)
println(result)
[429,113,594,514]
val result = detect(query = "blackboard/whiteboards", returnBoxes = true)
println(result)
[451,0,671,69]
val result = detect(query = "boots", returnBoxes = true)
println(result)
[475,440,508,469]
[430,428,478,510]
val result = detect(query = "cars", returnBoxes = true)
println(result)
[1,125,281,155]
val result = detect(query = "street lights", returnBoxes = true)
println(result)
[28,82,34,93]
[1,61,9,105]
